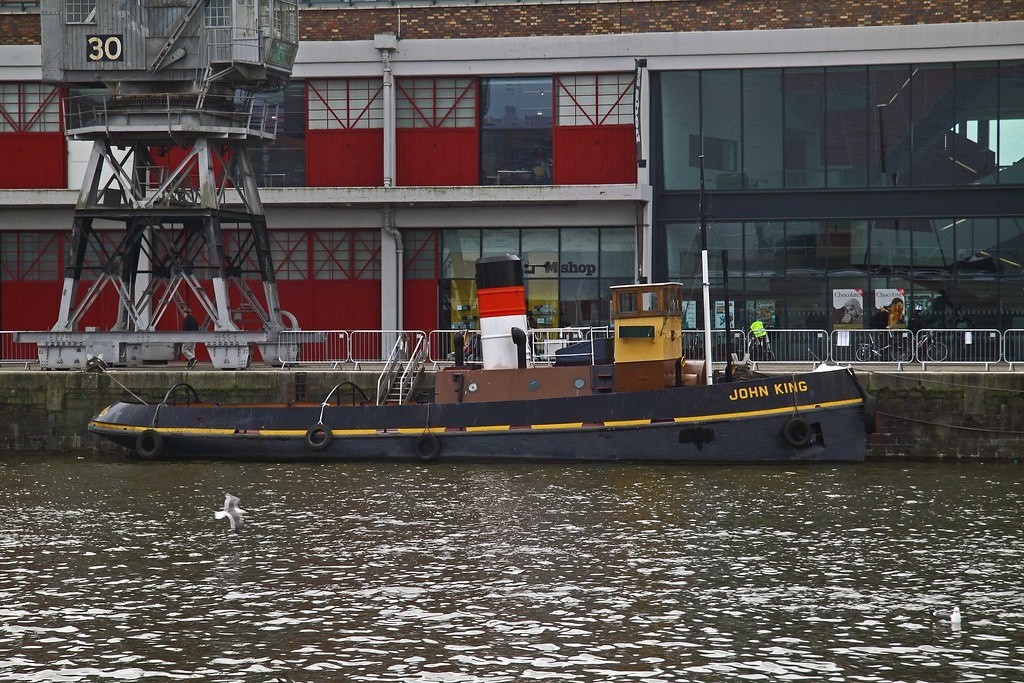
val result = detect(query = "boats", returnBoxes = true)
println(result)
[84,280,874,463]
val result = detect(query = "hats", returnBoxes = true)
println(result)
[845,299,863,316]
[884,298,903,309]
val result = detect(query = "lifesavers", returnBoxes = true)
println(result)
[781,415,813,451]
[305,422,335,451]
[412,431,443,462]
[135,428,165,458]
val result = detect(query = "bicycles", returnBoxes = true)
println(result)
[747,336,775,360]
[856,326,911,362]
[890,328,948,361]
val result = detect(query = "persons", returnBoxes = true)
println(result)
[181,309,200,369]
[878,307,890,361]
[805,303,826,360]
[842,299,863,324]
